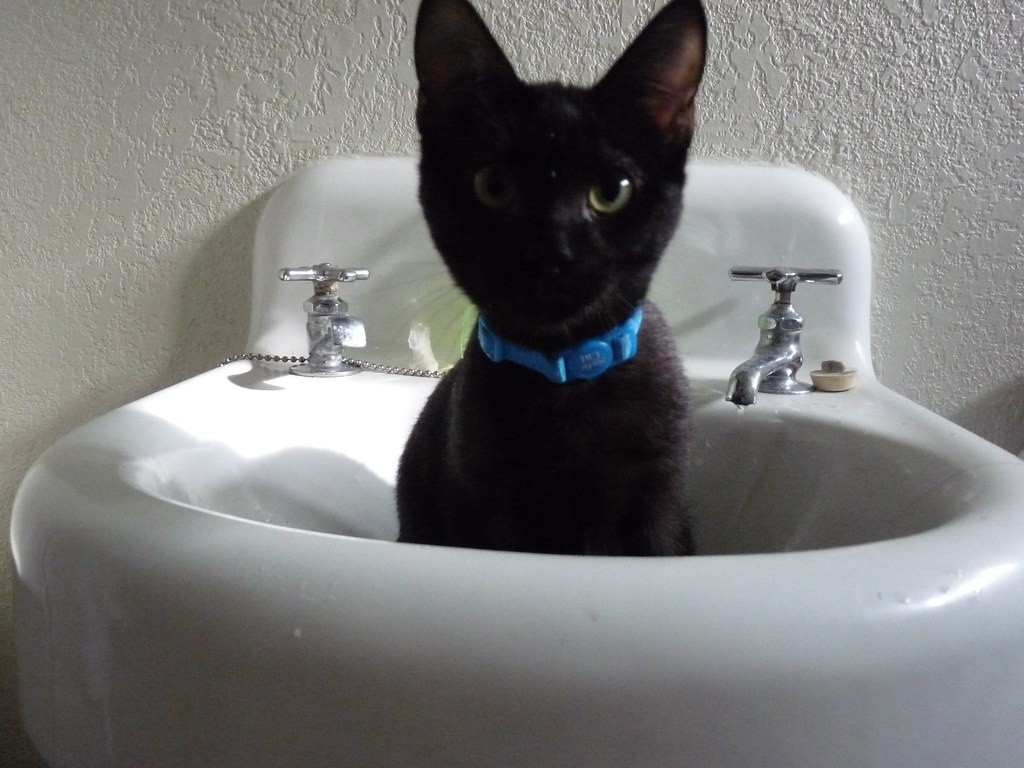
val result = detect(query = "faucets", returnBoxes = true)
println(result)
[277,263,372,378]
[723,263,842,406]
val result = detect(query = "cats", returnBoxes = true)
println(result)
[395,1,709,555]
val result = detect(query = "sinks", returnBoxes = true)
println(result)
[2,366,1024,768]
